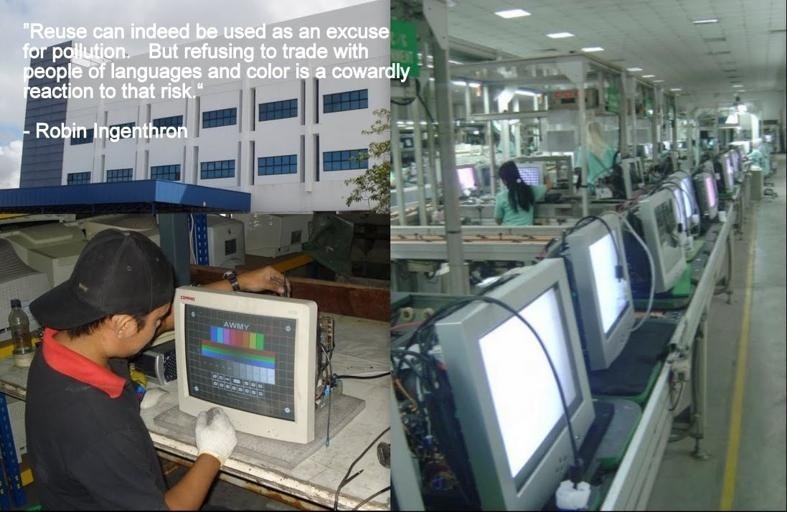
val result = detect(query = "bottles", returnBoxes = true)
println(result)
[8,299,32,354]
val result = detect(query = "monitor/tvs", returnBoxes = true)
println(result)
[171,285,336,445]
[420,135,772,512]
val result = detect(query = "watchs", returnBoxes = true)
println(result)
[222,269,242,292]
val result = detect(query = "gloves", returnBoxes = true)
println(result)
[194,406,238,470]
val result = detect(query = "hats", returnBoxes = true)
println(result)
[28,227,175,331]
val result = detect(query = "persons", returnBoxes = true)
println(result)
[756,138,770,159]
[23,227,293,512]
[493,158,555,226]
[577,121,617,182]
[744,141,770,177]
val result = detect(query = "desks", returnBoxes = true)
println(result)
[2,309,393,512]
